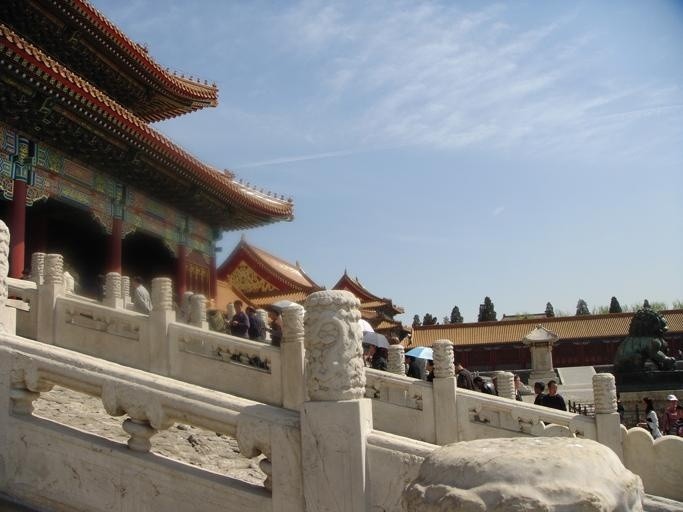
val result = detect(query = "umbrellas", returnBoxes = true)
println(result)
[269,299,306,321]
[359,317,375,333]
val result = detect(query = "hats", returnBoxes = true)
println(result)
[666,394,677,401]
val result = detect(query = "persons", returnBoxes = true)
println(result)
[20,269,32,281]
[543,380,567,426]
[667,403,683,439]
[62,264,74,294]
[663,394,678,435]
[637,397,664,441]
[170,293,186,322]
[128,275,153,315]
[532,381,546,406]
[205,298,283,370]
[363,332,523,426]
[615,393,626,420]
[94,273,105,304]
